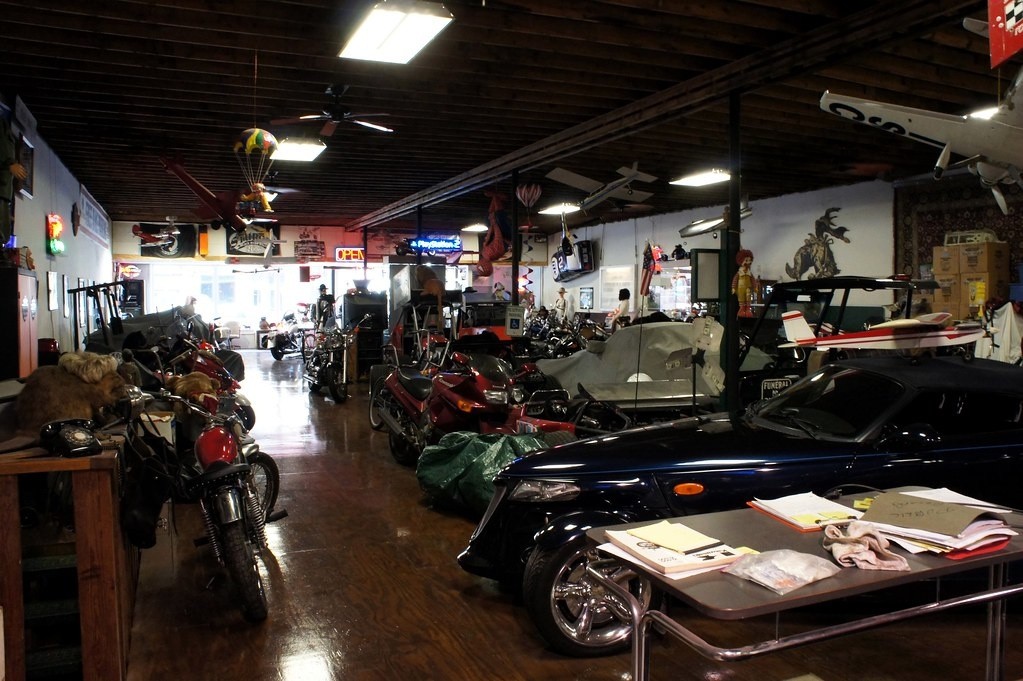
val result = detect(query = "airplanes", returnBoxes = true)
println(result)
[820,64,1023,193]
[132,222,181,251]
[678,206,757,245]
[546,162,658,216]
[778,307,987,360]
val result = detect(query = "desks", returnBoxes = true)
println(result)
[586,486,1023,681]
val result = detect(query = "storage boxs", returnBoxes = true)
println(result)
[933,241,1010,327]
[136,411,176,504]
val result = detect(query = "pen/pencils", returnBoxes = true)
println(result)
[816,516,857,523]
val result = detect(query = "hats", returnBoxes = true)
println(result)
[556,287,568,294]
[319,284,328,291]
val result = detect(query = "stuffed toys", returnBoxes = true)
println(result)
[494,282,505,301]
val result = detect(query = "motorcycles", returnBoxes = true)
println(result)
[262,316,303,358]
[371,350,536,469]
[130,308,256,430]
[297,324,354,403]
[183,416,282,622]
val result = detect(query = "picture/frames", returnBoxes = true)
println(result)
[47,271,115,336]
[15,131,36,200]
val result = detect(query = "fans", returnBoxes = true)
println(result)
[270,84,394,137]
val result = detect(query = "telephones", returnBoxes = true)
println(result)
[42,418,103,457]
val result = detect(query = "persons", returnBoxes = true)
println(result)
[523,304,533,335]
[730,249,756,317]
[553,287,568,324]
[240,183,271,215]
[608,289,630,324]
[117,348,142,389]
[317,284,335,330]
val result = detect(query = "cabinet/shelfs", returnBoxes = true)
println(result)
[0,411,139,681]
[0,266,38,383]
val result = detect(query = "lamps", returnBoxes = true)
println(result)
[268,134,328,163]
[460,217,489,232]
[199,225,209,256]
[666,166,743,188]
[538,195,581,215]
[335,0,456,66]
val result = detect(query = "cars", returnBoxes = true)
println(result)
[446,349,1021,658]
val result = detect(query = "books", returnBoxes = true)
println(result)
[603,520,743,573]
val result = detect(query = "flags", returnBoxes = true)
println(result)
[640,243,656,296]
